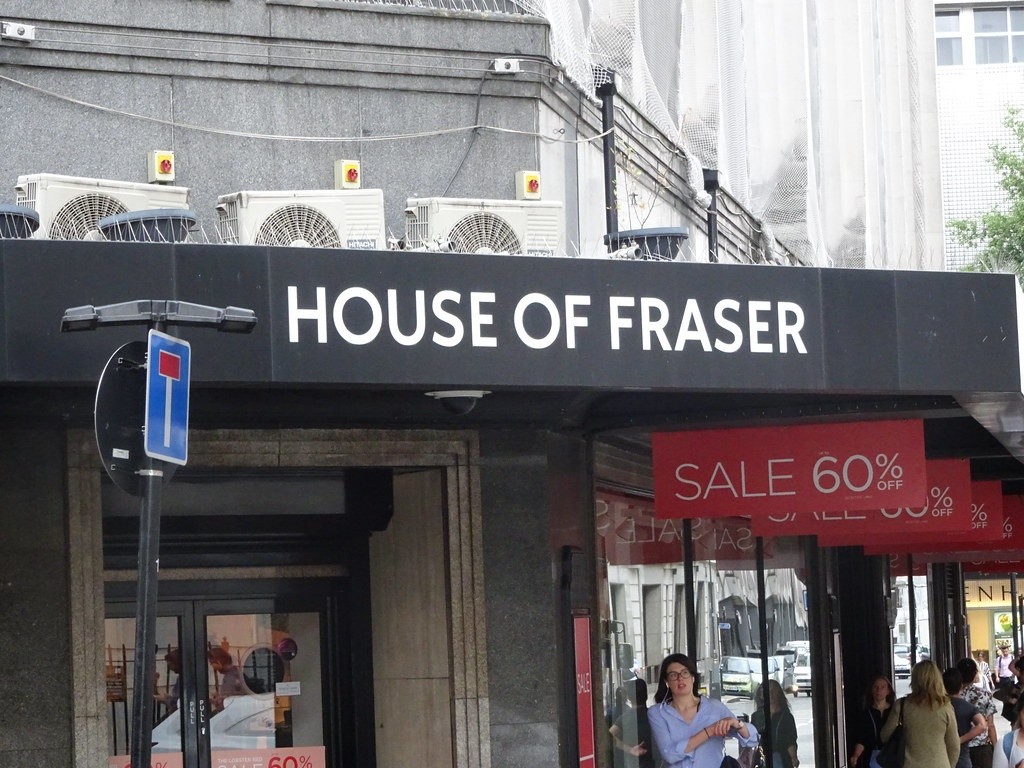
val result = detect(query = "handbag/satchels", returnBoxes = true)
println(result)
[876,697,907,768]
[720,755,741,768]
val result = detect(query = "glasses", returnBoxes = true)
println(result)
[667,668,691,680]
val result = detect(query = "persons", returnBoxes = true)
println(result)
[647,653,761,768]
[957,656,997,768]
[942,657,987,768]
[879,660,961,768]
[850,675,896,768]
[153,650,182,715]
[206,647,249,713]
[606,678,655,768]
[971,652,996,693]
[996,644,1024,768]
[751,679,799,768]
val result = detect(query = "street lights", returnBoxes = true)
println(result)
[60,300,261,768]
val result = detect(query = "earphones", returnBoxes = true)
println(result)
[692,676,694,682]
[665,682,669,688]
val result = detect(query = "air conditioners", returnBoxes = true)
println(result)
[217,189,386,250]
[404,198,566,256]
[17,173,187,239]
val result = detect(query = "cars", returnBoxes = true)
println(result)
[720,655,755,701]
[150,690,278,752]
[748,640,813,698]
[895,643,930,680]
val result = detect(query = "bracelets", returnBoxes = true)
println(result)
[704,728,710,738]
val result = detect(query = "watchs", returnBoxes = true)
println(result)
[736,720,743,731]
